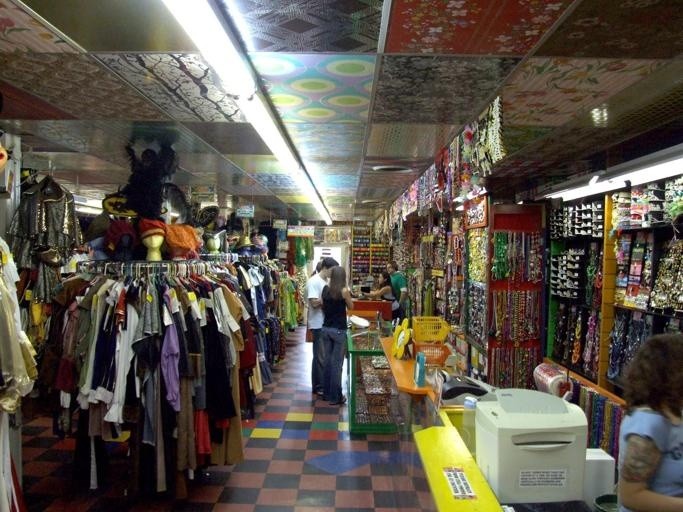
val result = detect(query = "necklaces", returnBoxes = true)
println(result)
[486,228,544,392]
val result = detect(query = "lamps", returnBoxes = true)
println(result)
[159,0,259,101]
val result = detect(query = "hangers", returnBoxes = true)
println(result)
[57,252,279,305]
[23,174,74,201]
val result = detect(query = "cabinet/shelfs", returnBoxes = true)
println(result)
[346,219,415,433]
[538,173,683,412]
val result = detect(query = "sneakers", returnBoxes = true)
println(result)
[313,390,347,406]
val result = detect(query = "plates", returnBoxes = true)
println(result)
[594,494,617,511]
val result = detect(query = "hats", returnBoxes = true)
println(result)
[137,218,167,241]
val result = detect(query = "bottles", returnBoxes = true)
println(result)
[375,310,382,330]
[462,396,476,456]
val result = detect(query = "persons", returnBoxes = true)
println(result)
[385,261,407,313]
[320,266,354,405]
[613,329,682,511]
[139,218,164,262]
[355,272,405,325]
[305,258,340,396]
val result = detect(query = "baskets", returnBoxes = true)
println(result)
[410,315,450,344]
[412,341,452,368]
